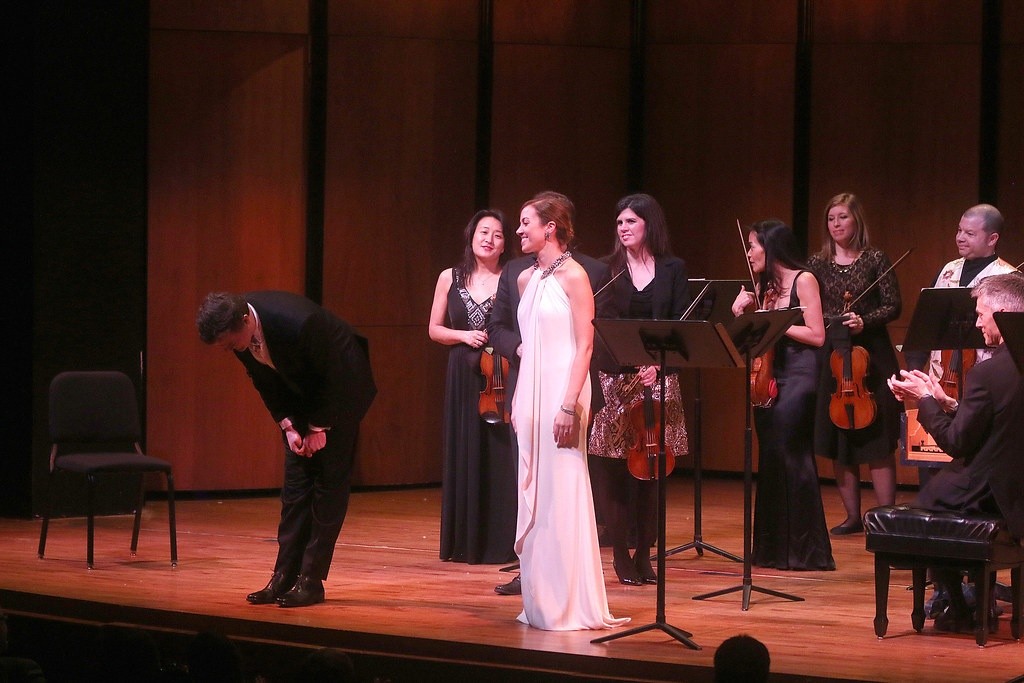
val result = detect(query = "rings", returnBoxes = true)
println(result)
[477,340,479,344]
[565,432,569,435]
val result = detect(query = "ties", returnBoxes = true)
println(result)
[251,337,261,351]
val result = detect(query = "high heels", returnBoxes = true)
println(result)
[613,560,642,586]
[634,555,659,584]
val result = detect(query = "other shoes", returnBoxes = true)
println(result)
[975,608,999,634]
[830,523,864,535]
[934,607,974,633]
[925,587,947,620]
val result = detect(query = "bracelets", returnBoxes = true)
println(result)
[560,405,576,416]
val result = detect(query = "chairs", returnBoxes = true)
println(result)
[37,370,179,568]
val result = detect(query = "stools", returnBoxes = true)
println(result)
[863,505,1024,648]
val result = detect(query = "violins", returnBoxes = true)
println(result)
[938,348,979,401]
[827,289,878,430]
[626,363,675,482]
[749,338,777,408]
[477,345,511,425]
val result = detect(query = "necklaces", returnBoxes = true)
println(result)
[534,251,572,278]
[477,273,493,285]
[831,254,861,273]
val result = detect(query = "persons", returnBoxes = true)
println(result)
[731,218,837,572]
[886,203,1024,634]
[587,194,697,586]
[807,192,902,535]
[488,190,613,595]
[513,198,632,631]
[428,209,520,566]
[196,290,378,607]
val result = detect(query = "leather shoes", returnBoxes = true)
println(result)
[246,571,297,602]
[276,576,325,606]
[495,577,522,595]
